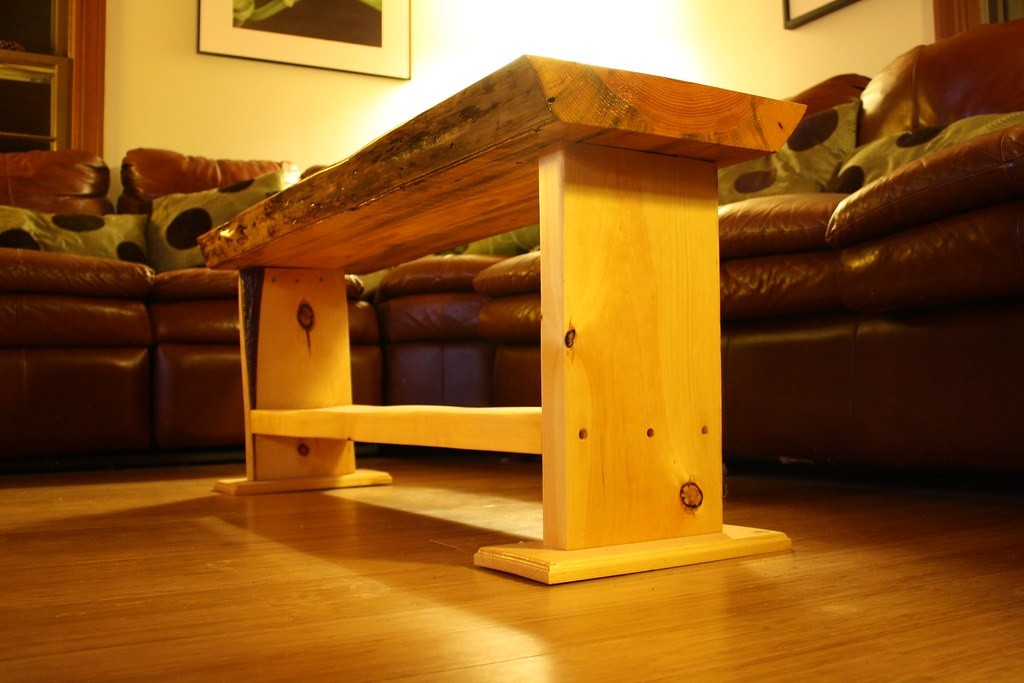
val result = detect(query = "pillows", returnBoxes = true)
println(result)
[827,111,1024,195]
[146,168,292,273]
[462,222,540,256]
[718,100,862,205]
[0,206,152,264]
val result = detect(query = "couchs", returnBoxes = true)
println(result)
[114,147,385,467]
[0,148,156,477]
[300,165,511,458]
[468,20,1024,511]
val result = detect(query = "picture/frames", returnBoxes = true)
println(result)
[782,0,861,30]
[196,0,413,80]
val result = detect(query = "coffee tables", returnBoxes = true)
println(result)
[195,54,808,587]
